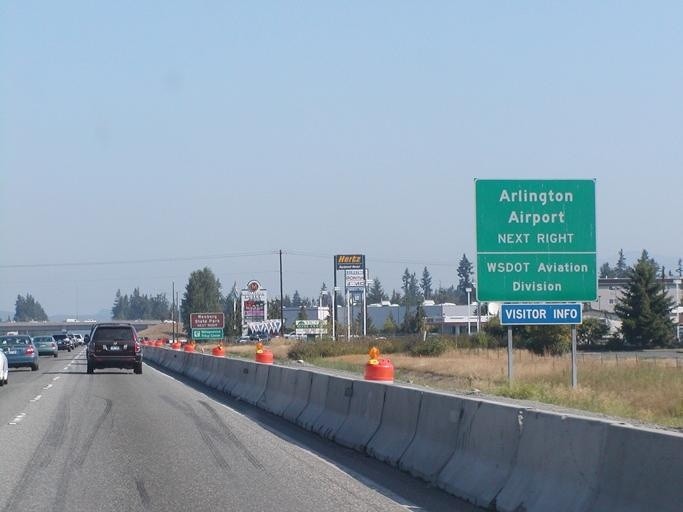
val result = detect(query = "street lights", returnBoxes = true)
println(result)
[318,285,342,342]
[464,286,472,334]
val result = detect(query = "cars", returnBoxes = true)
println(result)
[84,319,96,323]
[163,318,176,324]
[235,335,251,344]
[0,331,85,387]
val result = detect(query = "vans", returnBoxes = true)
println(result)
[65,318,80,322]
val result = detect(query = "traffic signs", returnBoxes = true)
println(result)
[189,312,224,328]
[475,177,599,303]
[192,329,223,339]
[502,304,583,325]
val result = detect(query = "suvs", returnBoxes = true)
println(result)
[83,322,143,375]
[282,330,308,340]
